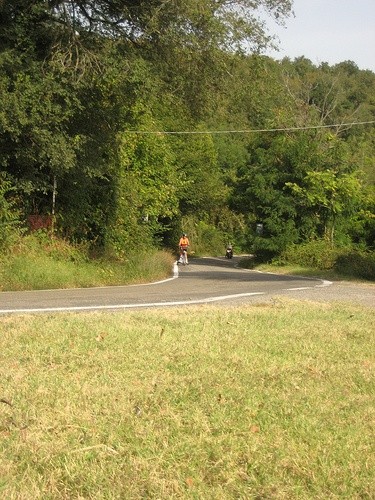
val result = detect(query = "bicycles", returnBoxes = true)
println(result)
[179,246,189,266]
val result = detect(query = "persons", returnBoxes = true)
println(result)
[177,233,191,265]
[227,242,233,250]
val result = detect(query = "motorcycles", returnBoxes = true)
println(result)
[226,249,232,259]
